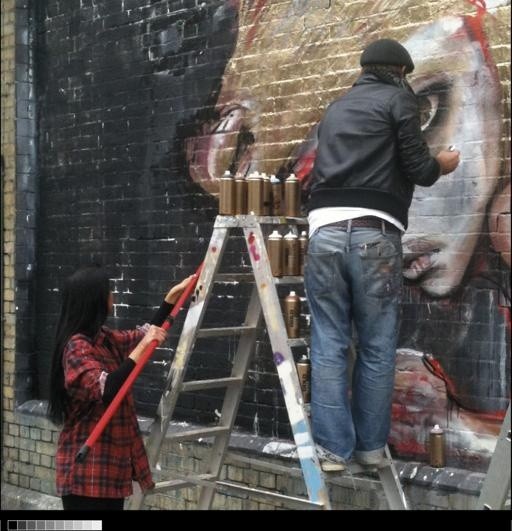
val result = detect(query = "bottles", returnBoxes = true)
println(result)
[428,422,446,467]
[217,166,302,216]
[266,227,311,403]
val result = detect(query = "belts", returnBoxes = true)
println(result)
[329,218,395,229]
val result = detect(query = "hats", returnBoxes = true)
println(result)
[361,40,416,73]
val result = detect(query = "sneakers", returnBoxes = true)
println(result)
[320,457,345,473]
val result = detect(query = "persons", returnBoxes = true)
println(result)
[45,265,197,511]
[298,39,461,475]
[181,2,511,473]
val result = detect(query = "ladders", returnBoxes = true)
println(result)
[130,214,409,510]
